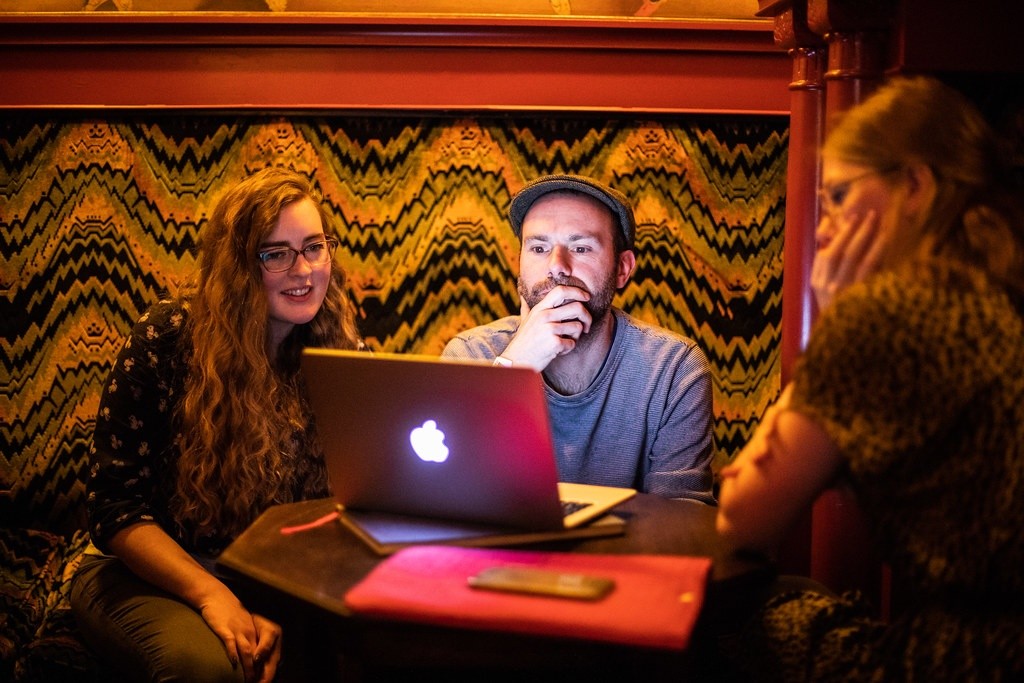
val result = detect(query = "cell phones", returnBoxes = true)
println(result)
[469,564,615,602]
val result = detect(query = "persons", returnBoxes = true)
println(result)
[714,76,1024,683]
[70,166,375,683]
[438,175,715,505]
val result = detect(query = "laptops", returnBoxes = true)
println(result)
[302,349,638,530]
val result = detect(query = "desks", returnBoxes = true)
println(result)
[218,484,759,627]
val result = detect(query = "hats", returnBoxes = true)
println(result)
[506,172,636,248]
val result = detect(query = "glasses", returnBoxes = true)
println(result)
[828,170,880,205]
[252,233,340,275]
